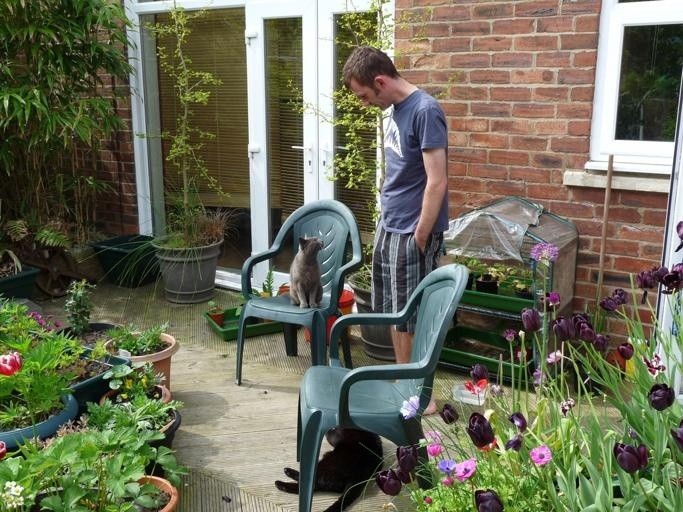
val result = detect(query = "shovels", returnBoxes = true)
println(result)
[573,154,614,398]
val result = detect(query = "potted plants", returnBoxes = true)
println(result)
[208,299,226,324]
[153,191,232,307]
[458,258,534,298]
[236,266,292,325]
[326,4,459,363]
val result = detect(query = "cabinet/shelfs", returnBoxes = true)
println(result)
[435,199,578,388]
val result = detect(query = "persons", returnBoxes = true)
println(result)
[339,45,451,418]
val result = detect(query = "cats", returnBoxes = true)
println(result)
[288,236,324,309]
[273,425,384,512]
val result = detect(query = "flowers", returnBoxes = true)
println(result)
[1,303,163,512]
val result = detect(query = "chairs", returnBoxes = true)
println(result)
[234,199,364,386]
[297,263,470,512]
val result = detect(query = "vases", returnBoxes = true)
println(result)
[104,333,178,388]
[91,234,153,286]
[1,260,39,297]
[97,387,179,449]
[127,472,180,512]
